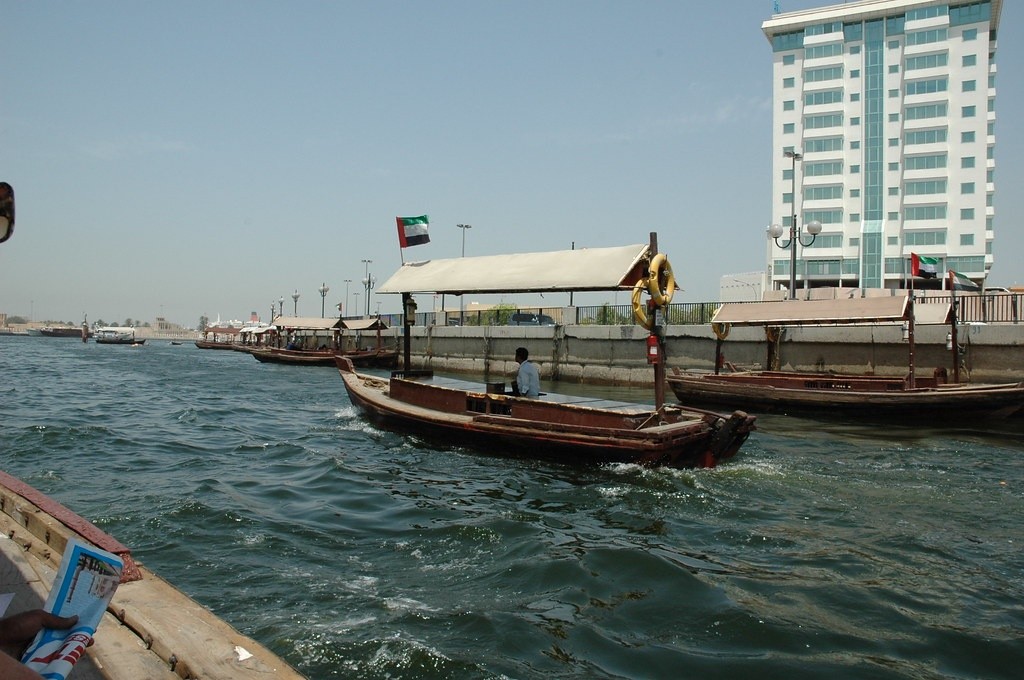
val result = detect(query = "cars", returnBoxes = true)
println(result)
[506,313,559,326]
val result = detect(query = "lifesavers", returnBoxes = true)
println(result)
[649,253,675,308]
[632,277,653,330]
[767,327,780,342]
[712,308,730,340]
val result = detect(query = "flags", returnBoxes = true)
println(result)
[951,271,980,292]
[396,215,430,247]
[911,253,939,278]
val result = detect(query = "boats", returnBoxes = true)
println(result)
[26,328,43,337]
[193,325,288,354]
[171,341,183,345]
[92,323,148,345]
[665,287,1023,429]
[336,232,757,471]
[251,315,400,369]
[40,326,93,338]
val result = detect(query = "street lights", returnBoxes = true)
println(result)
[279,295,285,317]
[353,292,360,316]
[457,223,472,326]
[362,273,376,314]
[784,149,803,300]
[344,280,353,317]
[271,299,276,322]
[292,289,301,317]
[764,212,824,300]
[318,283,330,318]
[361,258,374,315]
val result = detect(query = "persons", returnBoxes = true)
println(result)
[506,347,540,396]
[287,341,302,350]
[317,344,326,350]
[0,608,94,680]
